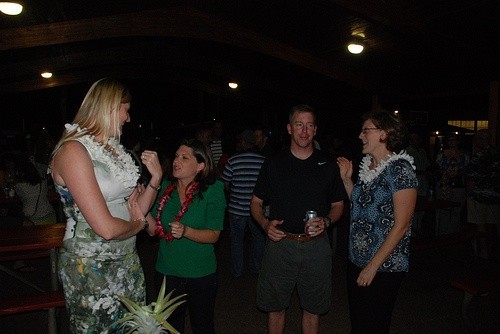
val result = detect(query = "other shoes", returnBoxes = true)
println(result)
[238,276,257,289]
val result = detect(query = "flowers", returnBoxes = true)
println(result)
[358,150,417,184]
[63,124,141,189]
[156,182,200,242]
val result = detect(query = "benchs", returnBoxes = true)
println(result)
[0,291,66,334]
[23,221,65,228]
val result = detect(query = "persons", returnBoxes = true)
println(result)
[0,153,57,270]
[250,104,346,334]
[51,77,163,334]
[136,138,227,334]
[320,127,500,261]
[195,120,272,180]
[222,130,269,288]
[336,106,418,334]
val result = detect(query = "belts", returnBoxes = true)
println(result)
[285,232,309,241]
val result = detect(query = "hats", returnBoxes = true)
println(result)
[236,130,256,144]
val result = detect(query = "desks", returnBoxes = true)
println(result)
[0,188,63,223]
[0,222,66,317]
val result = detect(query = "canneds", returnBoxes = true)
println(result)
[305,211,317,235]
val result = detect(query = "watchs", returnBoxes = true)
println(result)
[323,215,331,228]
[148,181,161,191]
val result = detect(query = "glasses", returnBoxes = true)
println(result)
[361,128,378,134]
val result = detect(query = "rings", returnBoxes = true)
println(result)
[143,155,147,160]
[318,227,321,231]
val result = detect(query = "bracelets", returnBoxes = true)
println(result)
[133,217,148,229]
[262,219,269,230]
